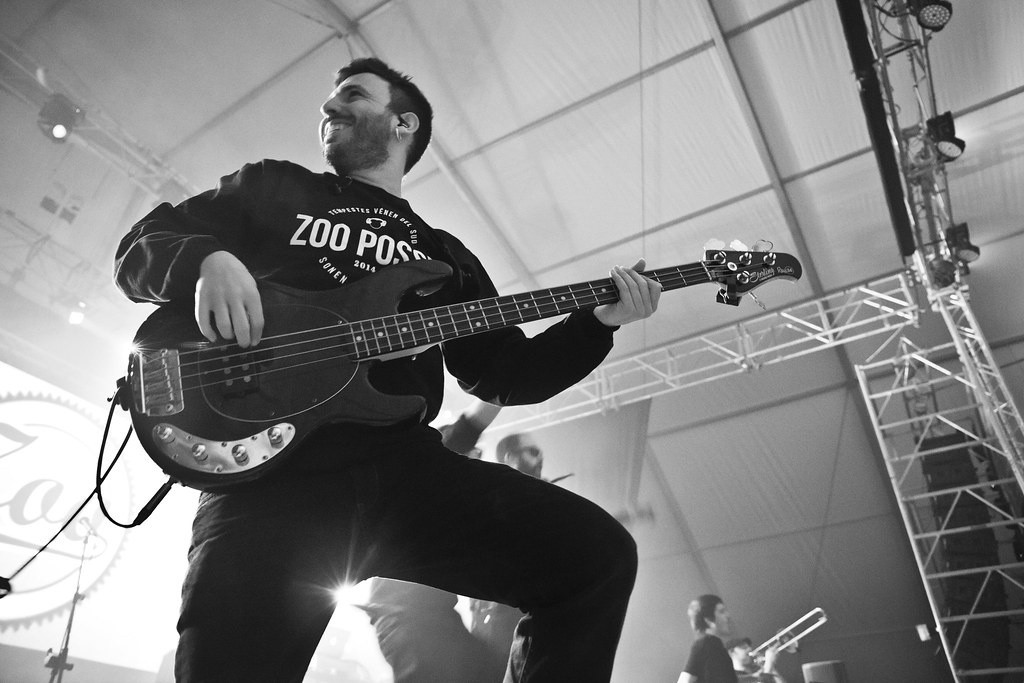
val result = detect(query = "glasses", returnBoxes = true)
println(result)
[524,447,539,456]
[474,447,483,458]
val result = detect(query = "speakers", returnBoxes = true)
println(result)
[916,429,1011,683]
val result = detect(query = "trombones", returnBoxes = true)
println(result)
[749,605,828,665]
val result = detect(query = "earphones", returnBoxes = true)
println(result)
[397,117,409,128]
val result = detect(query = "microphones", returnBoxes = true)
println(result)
[79,516,96,536]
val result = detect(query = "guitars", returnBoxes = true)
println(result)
[127,238,803,495]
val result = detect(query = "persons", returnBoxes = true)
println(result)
[676,595,786,683]
[115,58,662,683]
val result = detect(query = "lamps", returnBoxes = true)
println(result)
[932,254,971,290]
[37,93,85,144]
[926,110,966,157]
[907,0,952,32]
[945,222,981,263]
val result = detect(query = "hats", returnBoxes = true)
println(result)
[727,637,751,649]
